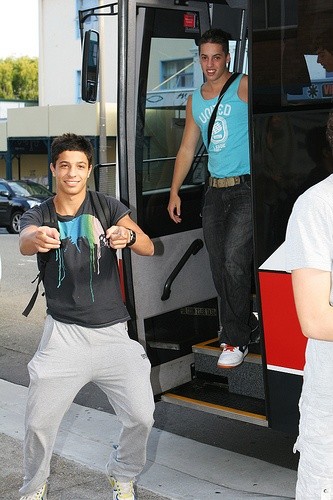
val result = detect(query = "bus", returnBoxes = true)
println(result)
[78,0,333,433]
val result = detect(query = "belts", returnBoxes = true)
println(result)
[207,174,250,188]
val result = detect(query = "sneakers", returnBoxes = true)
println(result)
[20,480,48,500]
[107,475,136,499]
[217,345,249,368]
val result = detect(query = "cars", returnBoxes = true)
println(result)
[0,178,56,234]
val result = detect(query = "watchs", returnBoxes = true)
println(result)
[126,228,136,247]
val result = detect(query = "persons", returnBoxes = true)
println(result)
[19,133,156,499]
[285,114,333,500]
[252,113,333,250]
[315,32,332,72]
[167,29,261,368]
